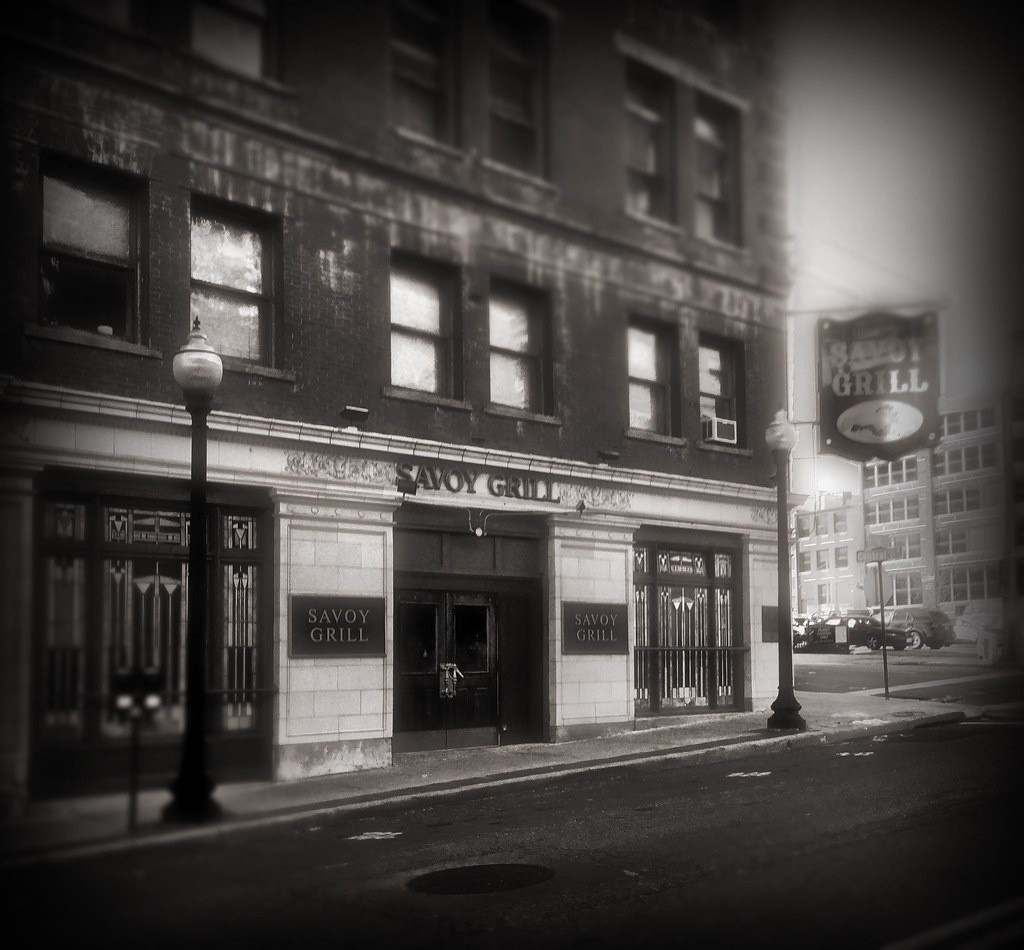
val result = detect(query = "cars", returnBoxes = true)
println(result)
[794,607,956,650]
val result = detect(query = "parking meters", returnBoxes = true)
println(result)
[115,683,165,831]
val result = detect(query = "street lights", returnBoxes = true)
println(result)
[765,406,808,729]
[156,314,224,827]
